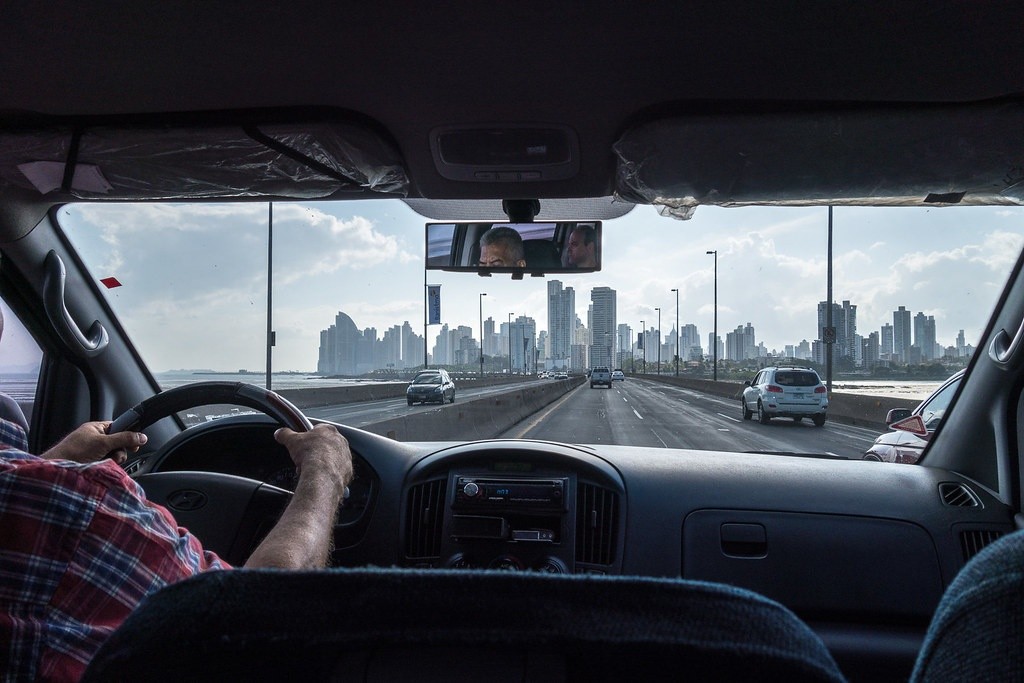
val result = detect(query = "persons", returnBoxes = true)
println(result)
[568,225,596,267]
[479,227,526,267]
[0,308,353,683]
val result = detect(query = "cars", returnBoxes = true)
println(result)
[512,370,568,379]
[406,373,456,406]
[614,369,622,371]
[611,371,624,381]
[861,367,968,465]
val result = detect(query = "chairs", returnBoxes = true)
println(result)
[561,247,578,269]
[78,566,848,682]
[908,530,1024,683]
[523,238,562,269]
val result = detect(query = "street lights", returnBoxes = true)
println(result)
[629,328,633,374]
[508,312,514,374]
[655,307,660,374]
[479,293,487,378]
[640,320,646,374]
[706,251,717,381]
[520,324,526,375]
[619,334,622,369]
[671,289,679,378]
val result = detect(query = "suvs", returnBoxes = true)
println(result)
[407,368,451,388]
[741,365,829,427]
[590,366,612,389]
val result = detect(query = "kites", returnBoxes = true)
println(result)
[100,277,122,288]
